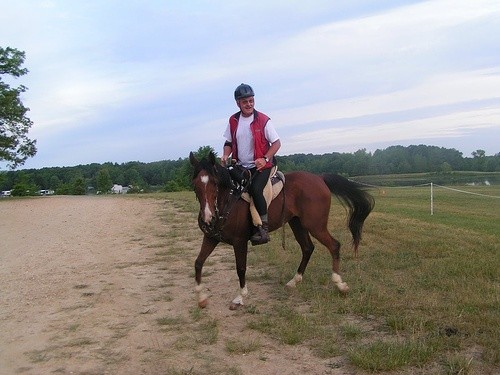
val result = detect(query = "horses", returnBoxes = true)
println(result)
[189,151,375,311]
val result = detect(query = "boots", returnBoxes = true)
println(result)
[251,224,270,241]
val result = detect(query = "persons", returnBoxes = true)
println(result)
[221,83,281,242]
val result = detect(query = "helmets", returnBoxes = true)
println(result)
[234,83,255,100]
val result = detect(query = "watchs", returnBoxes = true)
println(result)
[264,156,270,163]
